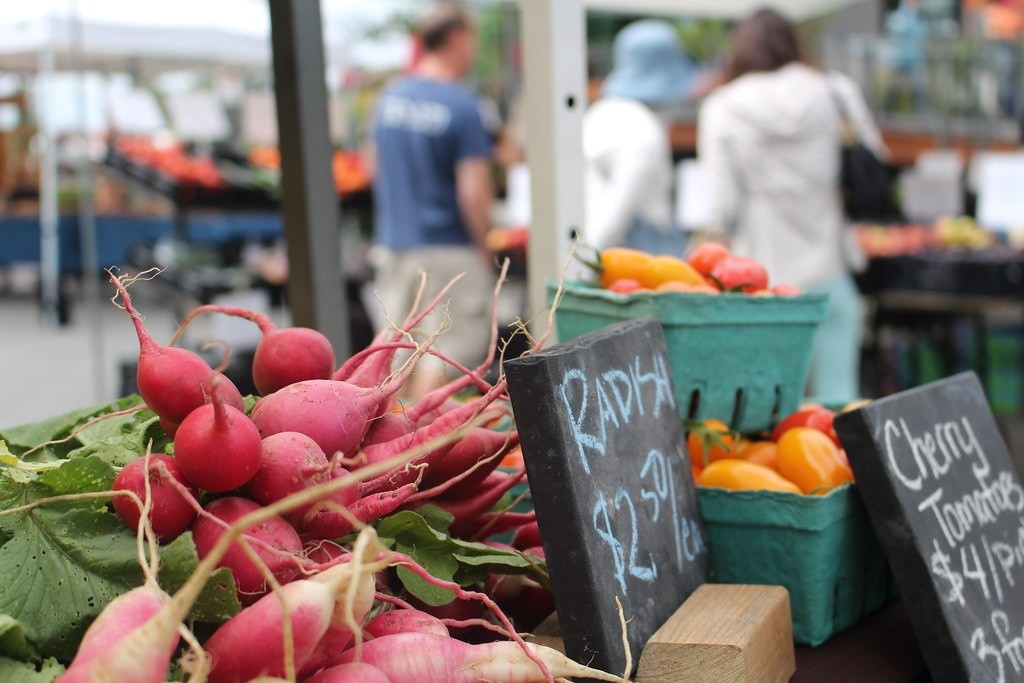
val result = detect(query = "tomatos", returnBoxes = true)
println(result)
[573,241,805,295]
[683,400,871,496]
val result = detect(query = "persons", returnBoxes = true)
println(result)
[372,1,492,395]
[685,9,893,402]
[581,19,693,262]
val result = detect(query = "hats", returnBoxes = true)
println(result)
[597,17,697,105]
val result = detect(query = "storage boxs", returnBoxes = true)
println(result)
[545,276,830,435]
[510,480,896,650]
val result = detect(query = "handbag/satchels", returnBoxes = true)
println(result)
[840,142,914,294]
